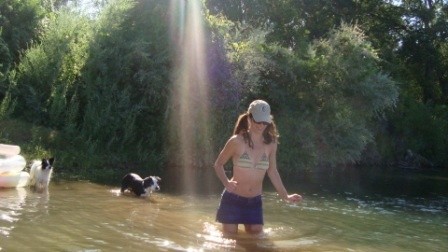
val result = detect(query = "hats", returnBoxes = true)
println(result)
[248,99,272,123]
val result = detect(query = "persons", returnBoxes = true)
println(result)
[214,101,303,238]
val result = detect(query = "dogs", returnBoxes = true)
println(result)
[120,173,162,198]
[29,157,56,191]
